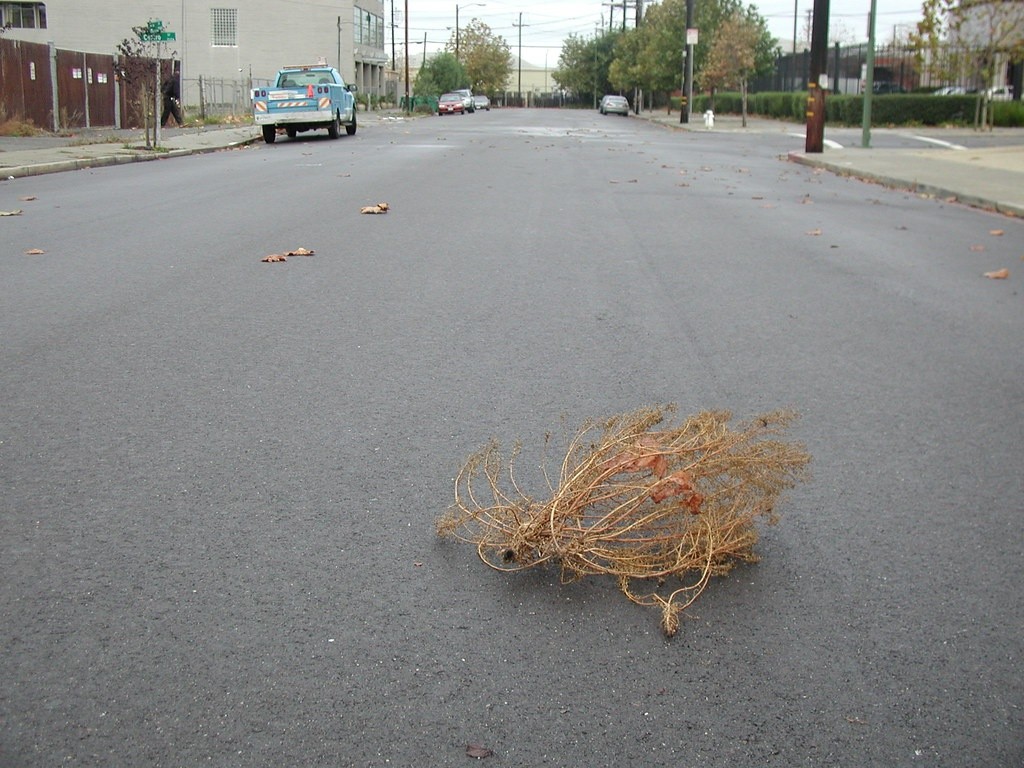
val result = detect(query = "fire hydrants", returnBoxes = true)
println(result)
[703,109,715,130]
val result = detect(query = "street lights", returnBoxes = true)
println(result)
[456,1,487,55]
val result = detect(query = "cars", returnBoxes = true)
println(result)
[935,84,1015,103]
[472,95,491,111]
[435,93,465,116]
[873,81,908,95]
[599,95,630,117]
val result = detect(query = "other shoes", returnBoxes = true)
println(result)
[179,123,188,128]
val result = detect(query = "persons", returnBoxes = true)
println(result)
[161,70,189,128]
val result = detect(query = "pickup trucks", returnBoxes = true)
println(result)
[250,62,358,143]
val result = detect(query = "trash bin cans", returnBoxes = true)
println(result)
[415,95,439,112]
[400,96,415,112]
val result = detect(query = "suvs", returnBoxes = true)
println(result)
[452,90,475,113]
